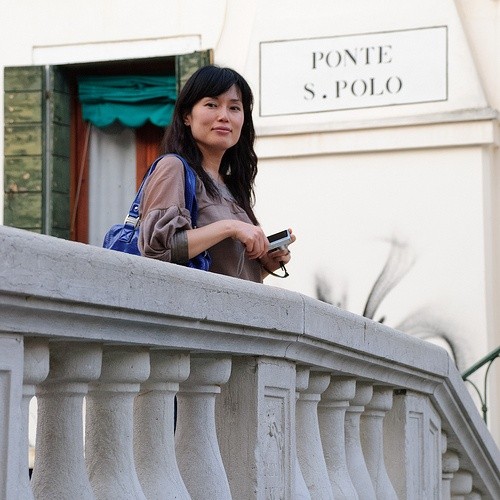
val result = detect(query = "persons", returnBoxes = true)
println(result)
[134,65,297,284]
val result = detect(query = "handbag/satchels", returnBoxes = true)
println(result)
[103,154,212,272]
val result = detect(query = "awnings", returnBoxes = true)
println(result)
[78,75,178,129]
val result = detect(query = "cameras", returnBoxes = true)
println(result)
[267,229,292,250]
